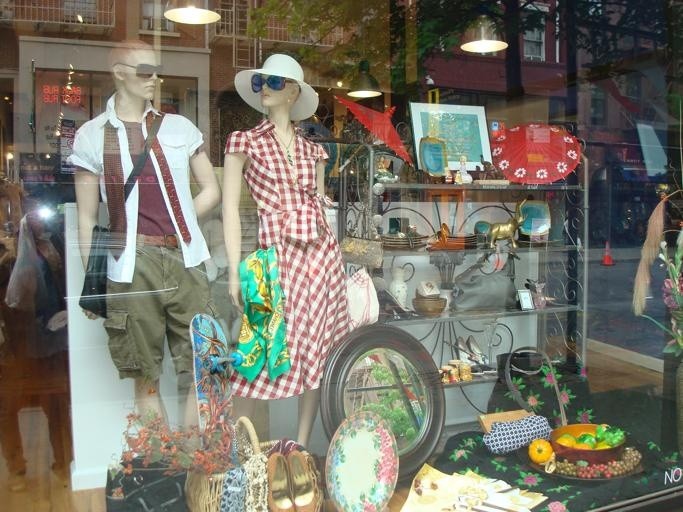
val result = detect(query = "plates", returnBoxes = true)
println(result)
[443,232,479,247]
[322,410,399,511]
[381,233,429,251]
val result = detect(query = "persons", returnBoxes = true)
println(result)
[222,53,349,454]
[66,38,222,444]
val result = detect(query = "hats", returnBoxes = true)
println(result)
[234,54,319,122]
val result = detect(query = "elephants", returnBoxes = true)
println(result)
[489,196,529,250]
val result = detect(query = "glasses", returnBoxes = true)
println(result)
[112,63,163,79]
[250,75,297,93]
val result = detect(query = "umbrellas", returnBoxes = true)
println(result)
[333,95,413,173]
[492,122,581,184]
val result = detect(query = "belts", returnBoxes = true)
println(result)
[137,234,182,249]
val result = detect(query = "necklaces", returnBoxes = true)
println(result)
[272,129,296,166]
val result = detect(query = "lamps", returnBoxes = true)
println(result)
[457,1,510,53]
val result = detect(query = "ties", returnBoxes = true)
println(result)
[103,111,191,262]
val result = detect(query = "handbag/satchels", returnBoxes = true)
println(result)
[79,225,109,319]
[487,350,593,429]
[105,457,187,512]
[184,416,284,512]
[339,236,384,269]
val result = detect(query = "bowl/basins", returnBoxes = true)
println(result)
[413,288,449,320]
[549,422,626,466]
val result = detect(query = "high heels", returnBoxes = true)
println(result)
[267,450,318,511]
[454,335,496,373]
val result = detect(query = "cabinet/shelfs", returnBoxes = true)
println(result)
[340,142,587,382]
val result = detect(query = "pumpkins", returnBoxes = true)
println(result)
[528,439,554,463]
